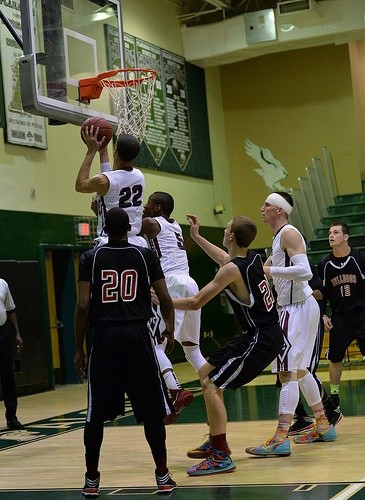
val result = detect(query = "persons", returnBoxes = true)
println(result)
[0,278,24,428]
[272,233,344,436]
[73,207,178,497]
[315,219,365,428]
[90,191,208,424]
[151,214,284,476]
[245,191,337,457]
[74,125,149,248]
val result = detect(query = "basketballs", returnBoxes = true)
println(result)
[80,116,113,149]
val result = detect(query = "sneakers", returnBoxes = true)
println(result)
[186,448,236,476]
[187,434,230,458]
[244,434,292,456]
[154,468,177,494]
[164,388,194,426]
[81,471,101,498]
[293,423,337,443]
[325,405,344,428]
[287,416,314,436]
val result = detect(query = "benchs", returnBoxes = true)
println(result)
[306,193,365,360]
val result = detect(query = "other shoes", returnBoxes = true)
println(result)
[6,416,25,429]
[326,394,341,410]
